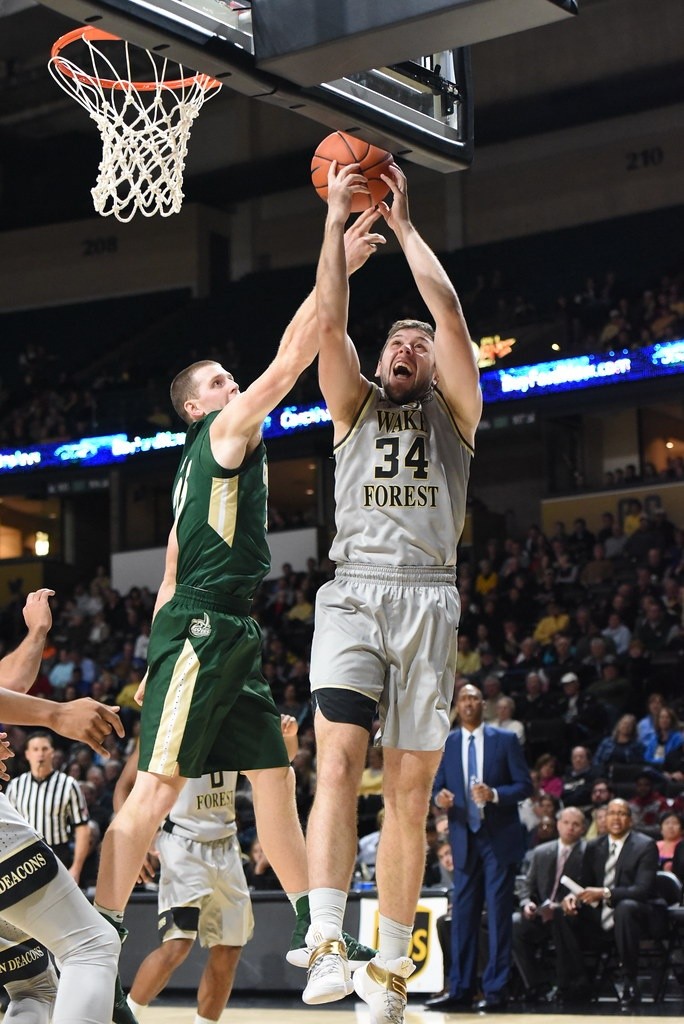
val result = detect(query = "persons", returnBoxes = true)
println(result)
[0,588,125,1024]
[93,207,388,1024]
[422,454,684,1016]
[0,733,15,790]
[236,556,386,892]
[0,560,158,853]
[301,159,483,1024]
[113,711,299,1024]
[4,732,90,886]
[0,266,684,447]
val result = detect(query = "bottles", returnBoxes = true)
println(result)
[352,872,362,894]
[470,774,484,808]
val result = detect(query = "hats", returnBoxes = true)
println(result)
[560,671,579,685]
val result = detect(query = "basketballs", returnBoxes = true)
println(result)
[310,130,396,214]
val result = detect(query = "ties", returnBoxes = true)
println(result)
[466,735,482,834]
[541,849,569,923]
[601,843,618,931]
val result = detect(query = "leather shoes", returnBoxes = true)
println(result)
[425,994,473,1013]
[470,996,508,1014]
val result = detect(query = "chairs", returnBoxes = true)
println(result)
[510,870,684,1024]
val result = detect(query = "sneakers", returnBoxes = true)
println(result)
[52,912,138,1024]
[302,922,355,1004]
[353,952,416,1024]
[287,895,378,970]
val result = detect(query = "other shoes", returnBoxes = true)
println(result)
[510,986,539,1004]
[559,983,599,1004]
[619,985,641,1006]
